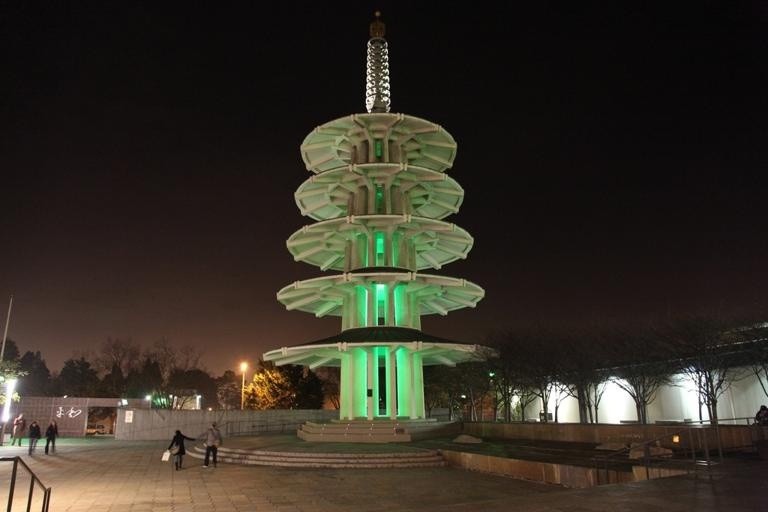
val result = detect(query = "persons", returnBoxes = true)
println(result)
[165,429,196,470]
[45,420,60,456]
[198,422,224,470]
[755,405,767,426]
[27,420,42,456]
[11,413,26,446]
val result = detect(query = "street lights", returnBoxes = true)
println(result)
[0,380,16,445]
[239,363,248,409]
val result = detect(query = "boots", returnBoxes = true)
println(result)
[175,461,181,470]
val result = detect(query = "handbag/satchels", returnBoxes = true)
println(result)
[161,446,179,461]
